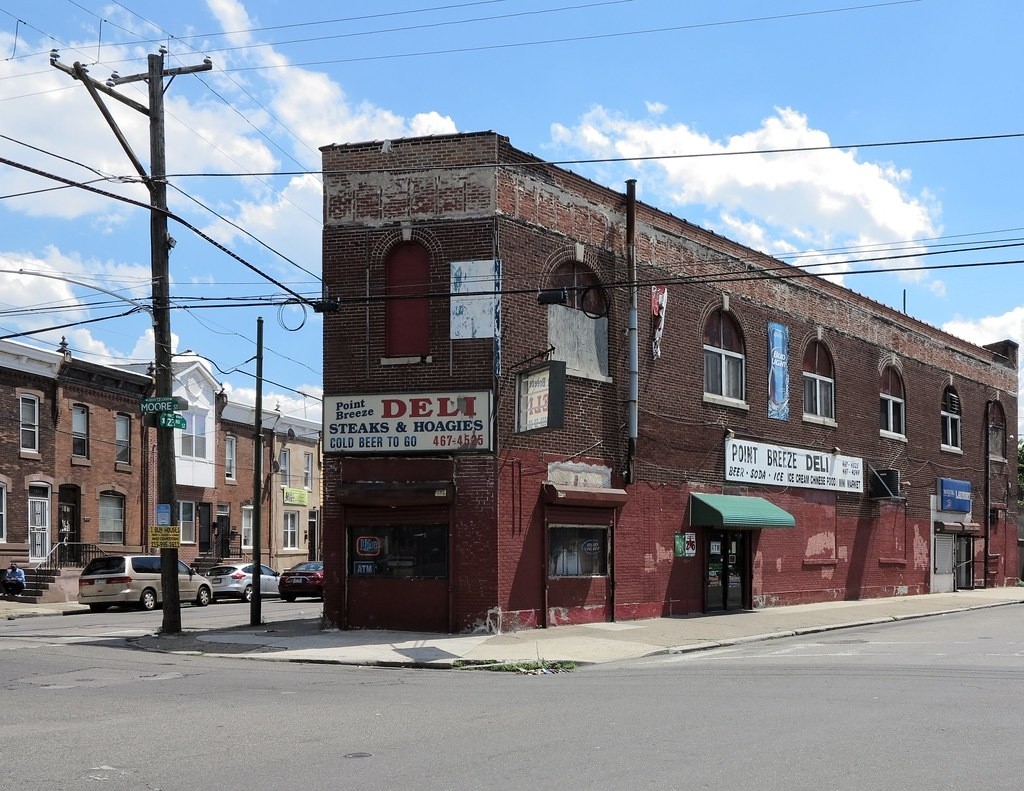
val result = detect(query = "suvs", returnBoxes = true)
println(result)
[78,553,213,612]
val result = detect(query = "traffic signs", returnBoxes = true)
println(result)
[160,412,187,429]
[139,396,180,412]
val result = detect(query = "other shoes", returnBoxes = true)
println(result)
[15,593,21,597]
[0,593,7,596]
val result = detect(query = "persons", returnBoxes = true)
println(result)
[0,563,26,597]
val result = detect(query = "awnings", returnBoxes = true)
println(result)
[690,492,796,527]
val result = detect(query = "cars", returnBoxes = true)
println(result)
[204,562,282,603]
[278,561,324,602]
